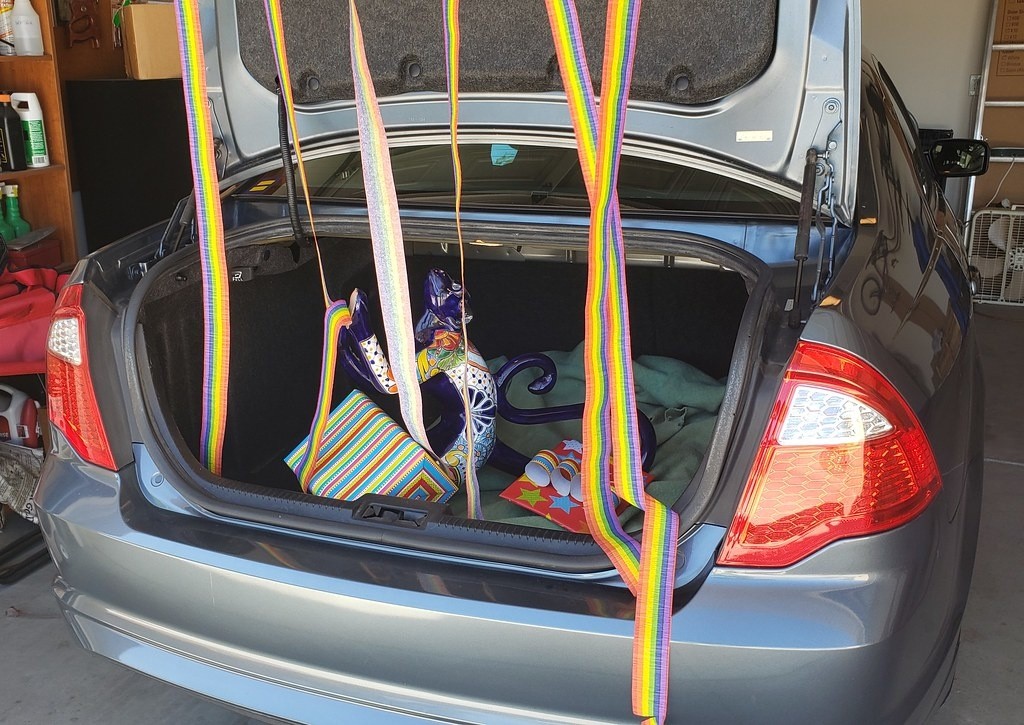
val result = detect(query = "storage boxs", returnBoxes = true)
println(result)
[283,389,459,520]
[8,239,62,267]
[118,4,182,80]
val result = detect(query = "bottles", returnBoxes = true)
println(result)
[5,185,30,238]
[0,187,15,241]
[10,0,44,57]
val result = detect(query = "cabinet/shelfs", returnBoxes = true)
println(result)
[0,0,78,276]
[962,0,1024,248]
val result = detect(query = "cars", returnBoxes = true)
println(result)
[29,0,991,724]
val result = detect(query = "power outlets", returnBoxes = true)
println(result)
[969,75,982,96]
